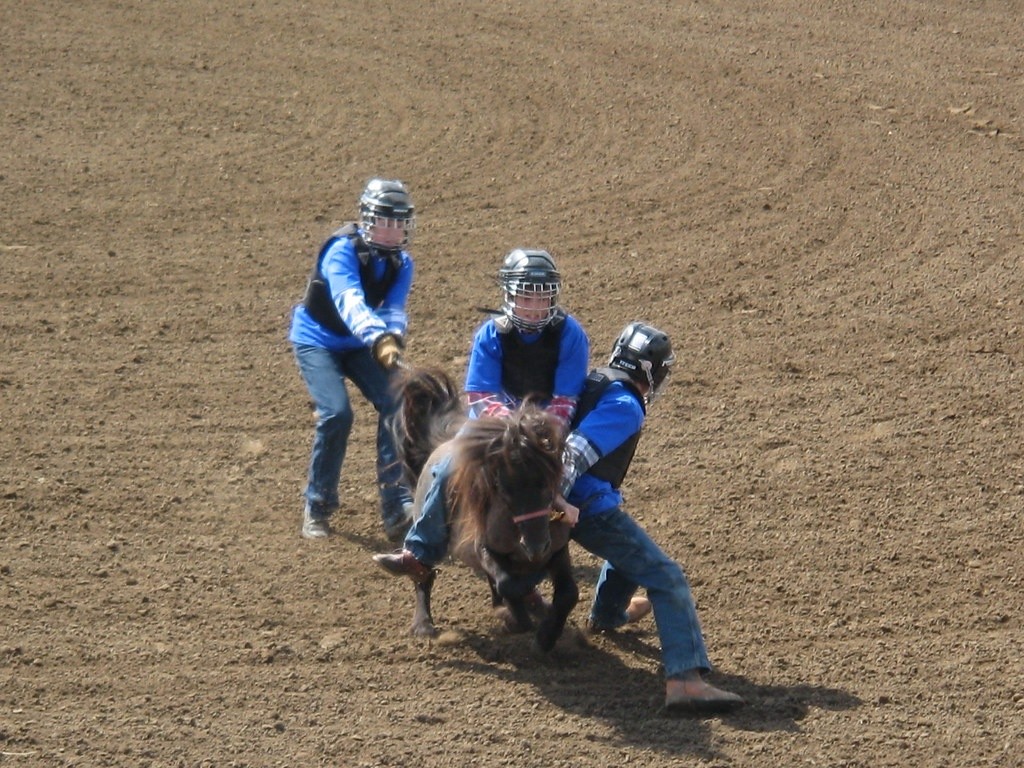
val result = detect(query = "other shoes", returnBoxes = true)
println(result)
[301,507,328,540]
[386,506,416,541]
[373,548,431,582]
[587,597,652,634]
[665,677,744,713]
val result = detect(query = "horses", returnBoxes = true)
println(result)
[380,361,579,659]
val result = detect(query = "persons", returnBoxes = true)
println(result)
[373,248,744,712]
[289,178,414,542]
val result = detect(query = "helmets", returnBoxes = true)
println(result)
[499,249,560,307]
[608,323,677,385]
[359,179,414,231]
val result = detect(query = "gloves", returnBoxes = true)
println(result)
[376,335,400,369]
